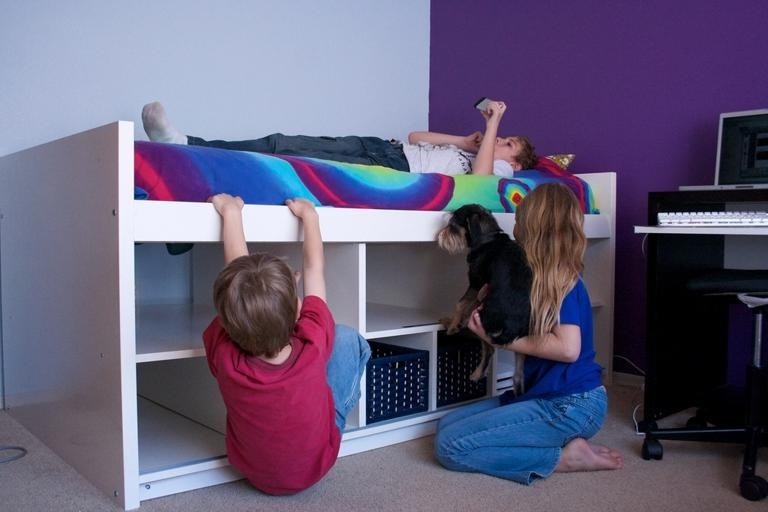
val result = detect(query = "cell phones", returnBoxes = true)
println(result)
[474,96,492,113]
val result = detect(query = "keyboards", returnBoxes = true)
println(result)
[656,211,768,228]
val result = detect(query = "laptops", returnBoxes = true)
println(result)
[679,108,767,190]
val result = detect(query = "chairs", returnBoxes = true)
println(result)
[636,270,766,503]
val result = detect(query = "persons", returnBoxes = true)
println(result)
[139,98,535,180]
[430,179,625,486]
[205,192,373,497]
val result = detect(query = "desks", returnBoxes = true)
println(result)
[643,190,768,419]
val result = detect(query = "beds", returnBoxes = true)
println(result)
[0,118,618,512]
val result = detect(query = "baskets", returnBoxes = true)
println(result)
[365,343,428,425]
[437,334,493,408]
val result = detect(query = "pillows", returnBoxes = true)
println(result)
[537,153,576,174]
